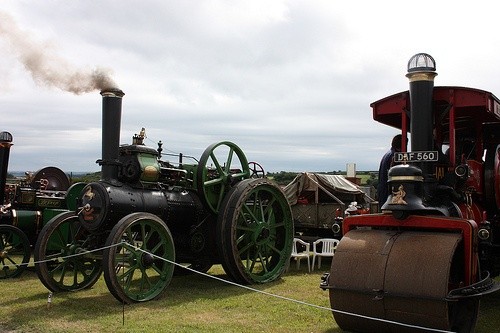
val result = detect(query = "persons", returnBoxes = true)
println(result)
[377,134,409,210]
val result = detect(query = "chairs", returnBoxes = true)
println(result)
[311,238,339,272]
[283,238,311,274]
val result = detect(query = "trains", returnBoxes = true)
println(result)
[320,53,500,333]
[1,87,295,306]
